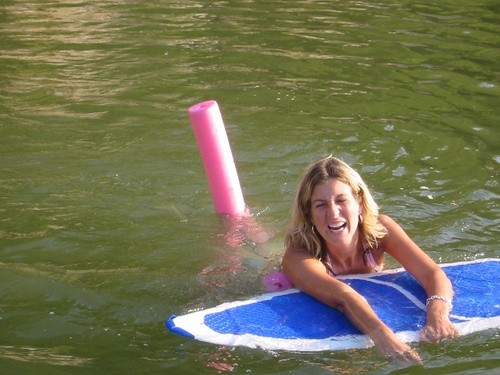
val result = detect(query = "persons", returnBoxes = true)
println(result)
[281,157,460,370]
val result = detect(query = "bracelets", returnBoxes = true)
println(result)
[425,295,453,311]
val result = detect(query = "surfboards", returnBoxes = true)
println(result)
[165,256,500,352]
